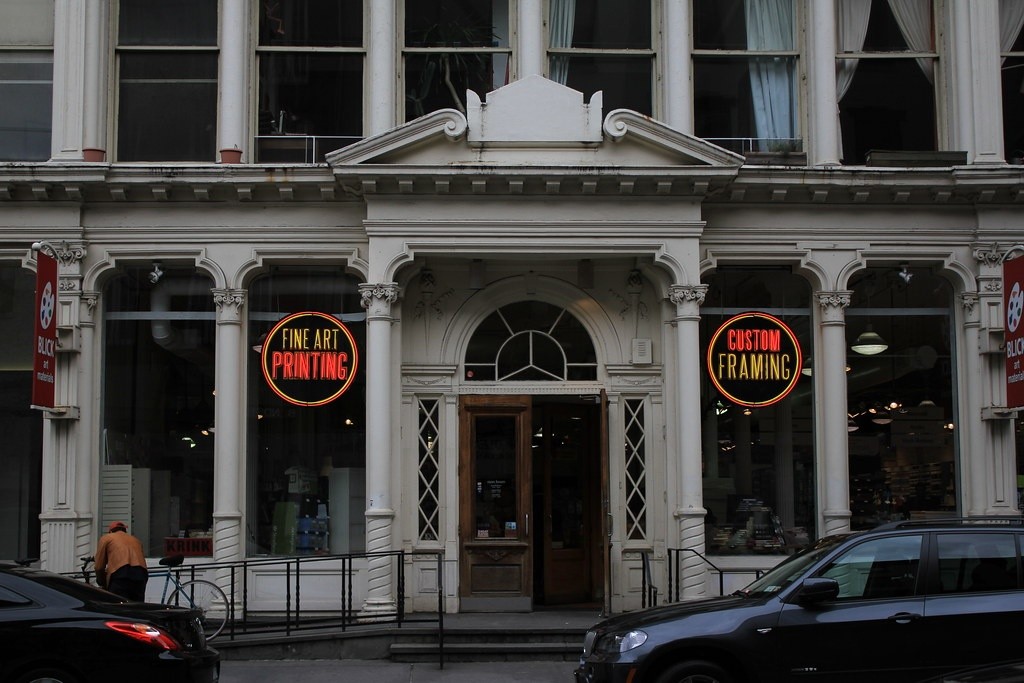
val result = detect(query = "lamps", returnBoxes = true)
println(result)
[252,323,271,353]
[851,316,888,355]
[801,358,812,376]
[898,260,915,284]
[469,259,486,290]
[578,259,594,288]
[847,403,893,431]
[148,262,163,284]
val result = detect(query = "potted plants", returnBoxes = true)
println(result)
[744,137,807,166]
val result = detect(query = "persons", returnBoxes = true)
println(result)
[95,522,148,602]
[863,546,893,597]
[967,543,1008,593]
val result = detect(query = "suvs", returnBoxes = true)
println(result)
[572,518,1024,683]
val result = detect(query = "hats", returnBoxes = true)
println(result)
[109,521,128,531]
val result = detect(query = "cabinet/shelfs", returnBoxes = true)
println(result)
[710,510,804,555]
[328,468,366,554]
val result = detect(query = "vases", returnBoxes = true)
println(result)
[219,150,243,164]
[864,150,968,167]
[82,149,106,162]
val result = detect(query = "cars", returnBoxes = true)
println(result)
[0,565,221,683]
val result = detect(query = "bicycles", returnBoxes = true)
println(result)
[81,554,229,642]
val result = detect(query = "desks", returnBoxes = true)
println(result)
[258,138,319,163]
